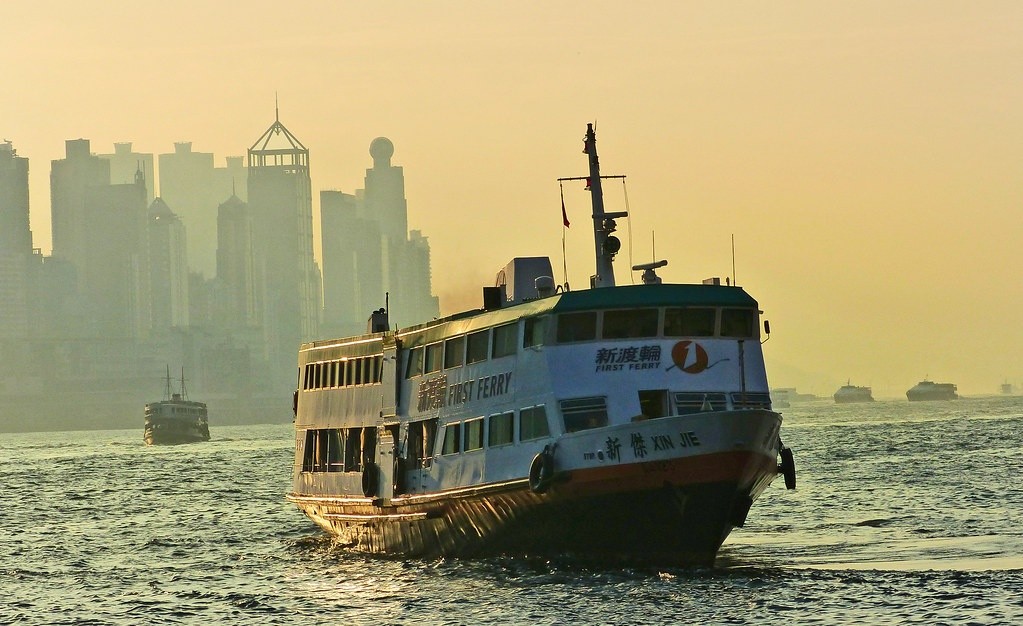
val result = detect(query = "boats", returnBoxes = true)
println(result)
[283,118,799,574]
[142,363,212,445]
[1002,383,1012,395]
[769,388,790,409]
[905,378,958,402]
[833,377,875,404]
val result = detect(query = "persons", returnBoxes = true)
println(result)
[583,413,599,429]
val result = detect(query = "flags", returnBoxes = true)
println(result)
[562,190,570,228]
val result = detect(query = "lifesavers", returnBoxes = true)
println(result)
[528,453,554,493]
[781,447,797,491]
[361,463,379,496]
[393,459,405,493]
